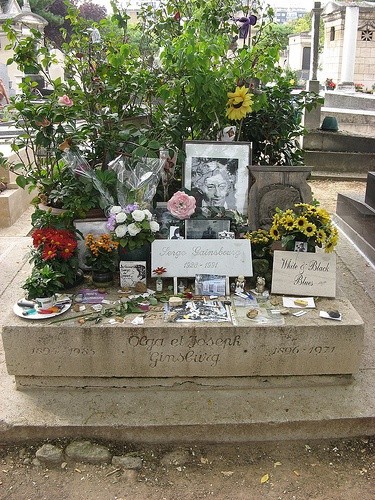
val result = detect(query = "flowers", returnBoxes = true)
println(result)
[20,0,375,299]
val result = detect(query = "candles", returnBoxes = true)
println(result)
[173,275,178,294]
[226,275,229,294]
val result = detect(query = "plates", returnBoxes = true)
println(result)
[12,294,71,319]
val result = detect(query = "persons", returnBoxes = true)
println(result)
[193,161,234,210]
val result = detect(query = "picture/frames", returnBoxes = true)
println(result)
[183,216,232,238]
[182,140,253,215]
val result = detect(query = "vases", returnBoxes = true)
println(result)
[156,275,163,293]
[92,270,113,287]
[117,244,146,261]
